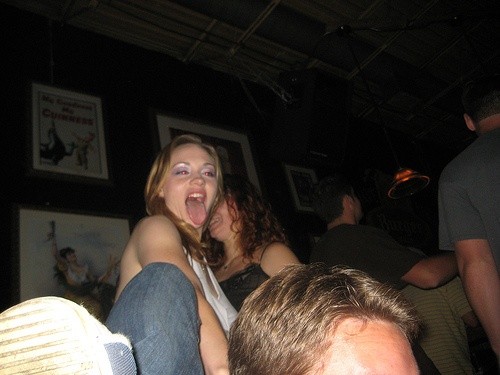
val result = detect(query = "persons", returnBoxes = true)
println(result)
[0,82,500,375]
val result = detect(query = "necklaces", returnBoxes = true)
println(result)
[224,256,236,271]
